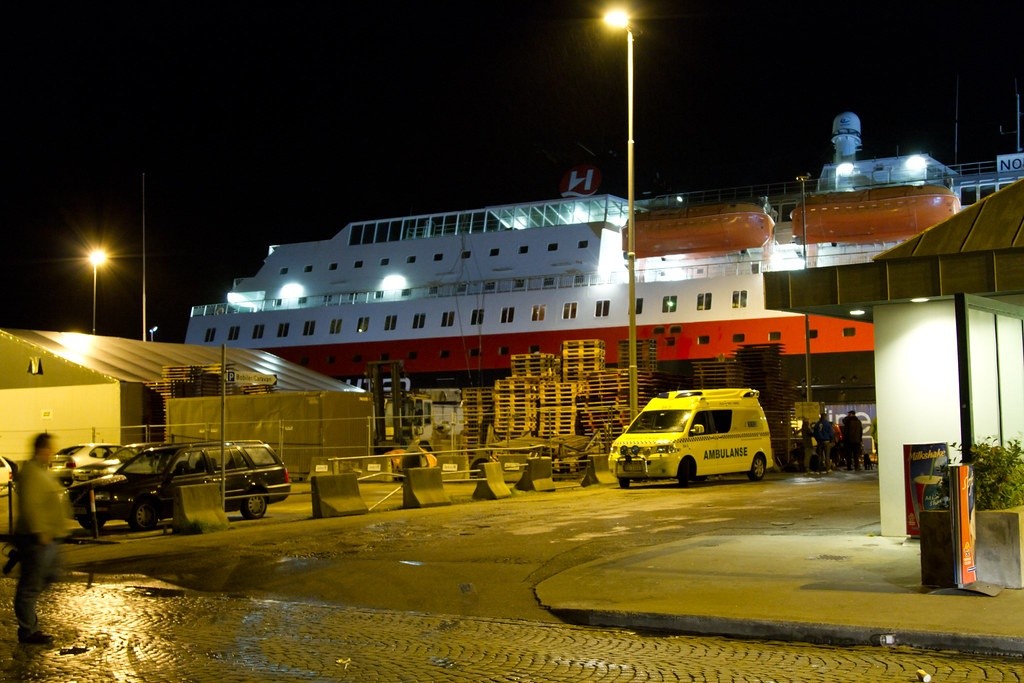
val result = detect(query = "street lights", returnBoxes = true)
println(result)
[88,249,104,336]
[601,5,639,424]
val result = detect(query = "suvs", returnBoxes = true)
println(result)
[61,438,291,534]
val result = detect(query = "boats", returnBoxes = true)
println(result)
[623,201,778,261]
[791,183,963,248]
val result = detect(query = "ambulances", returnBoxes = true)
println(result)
[606,388,775,490]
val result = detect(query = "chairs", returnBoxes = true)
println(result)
[171,456,238,476]
[70,452,109,458]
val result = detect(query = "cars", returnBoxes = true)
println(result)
[72,441,176,482]
[0,456,19,490]
[46,443,122,486]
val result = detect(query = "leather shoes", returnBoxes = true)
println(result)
[18,629,54,644]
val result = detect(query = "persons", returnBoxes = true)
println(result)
[813,414,836,472]
[802,421,815,474]
[13,432,70,645]
[841,411,864,472]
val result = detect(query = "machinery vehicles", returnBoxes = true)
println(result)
[364,356,436,459]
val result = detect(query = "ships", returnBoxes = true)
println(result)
[180,105,1023,436]
